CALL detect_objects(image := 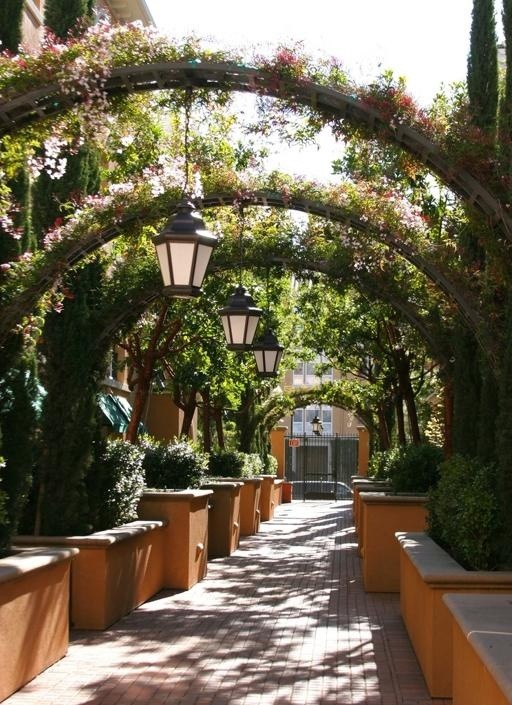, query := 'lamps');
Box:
[250,266,288,379]
[216,198,265,354]
[308,402,325,438]
[149,84,223,300]
